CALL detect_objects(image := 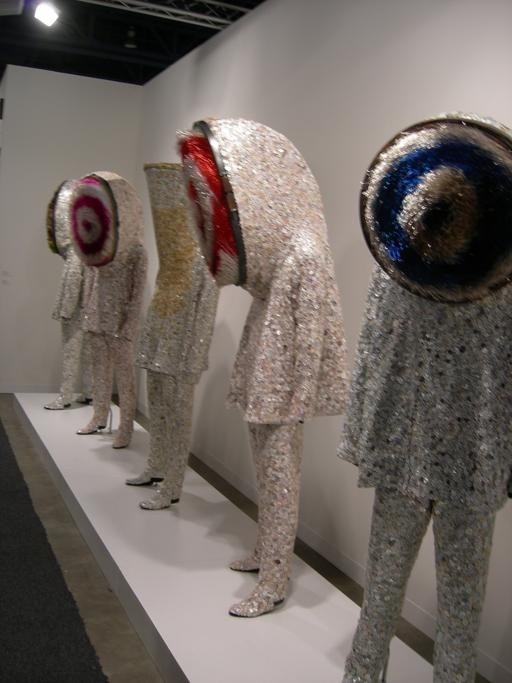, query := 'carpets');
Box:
[0,411,107,683]
